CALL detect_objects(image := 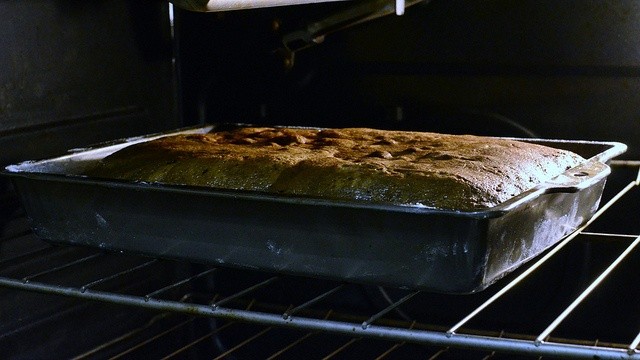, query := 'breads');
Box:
[92,124,587,213]
